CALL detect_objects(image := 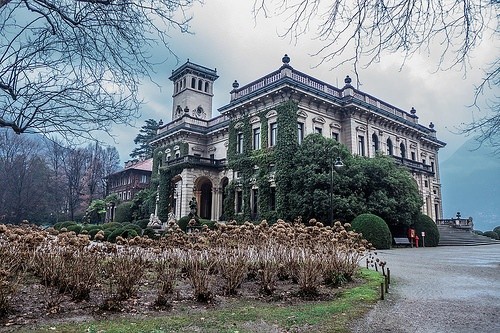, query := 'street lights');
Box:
[328,155,344,227]
